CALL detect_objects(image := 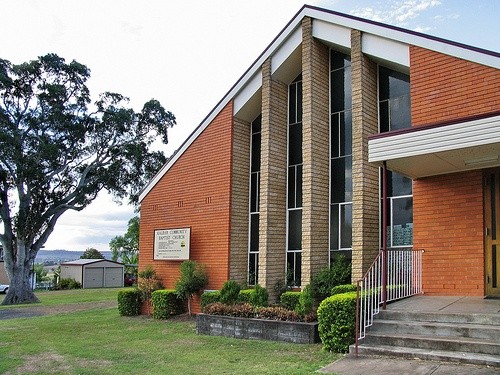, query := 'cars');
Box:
[124,273,135,287]
[0,283,9,295]
